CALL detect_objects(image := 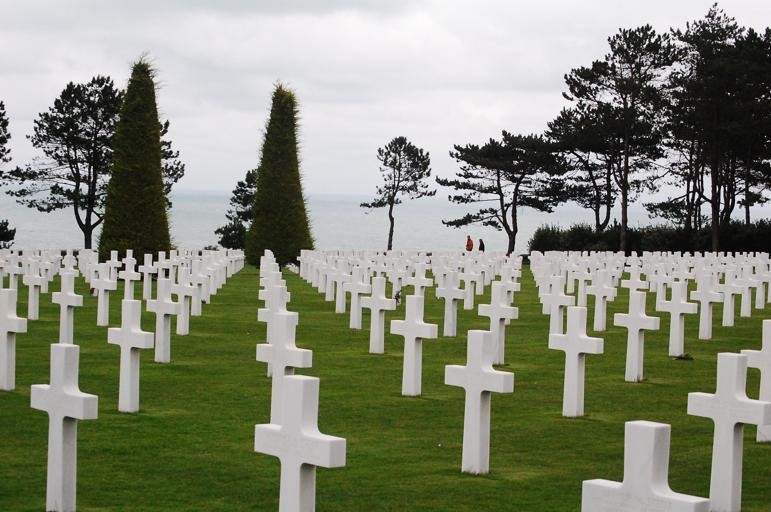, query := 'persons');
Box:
[478,239,484,250]
[465,235,475,251]
